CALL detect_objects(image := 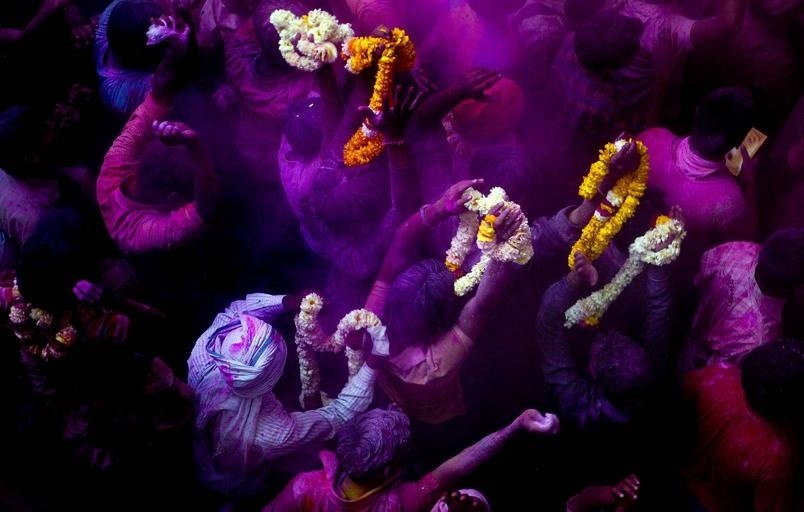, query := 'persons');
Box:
[1,2,802,512]
